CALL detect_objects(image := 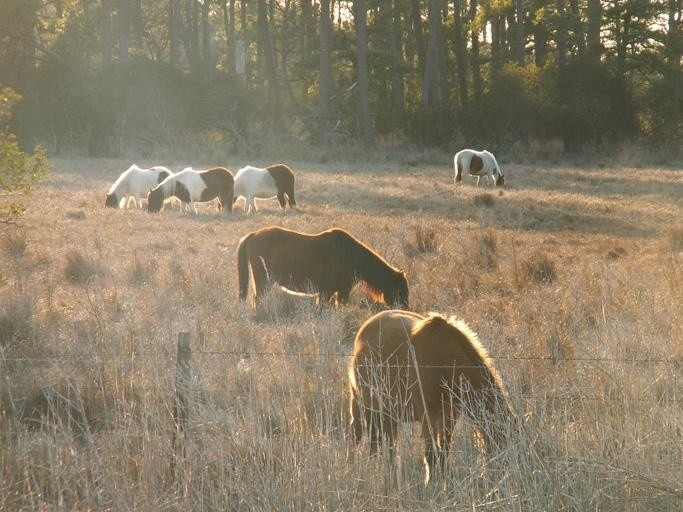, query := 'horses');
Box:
[350,308,533,488]
[104,164,173,209]
[147,167,235,216]
[453,148,505,189]
[218,164,297,215]
[236,225,409,315]
